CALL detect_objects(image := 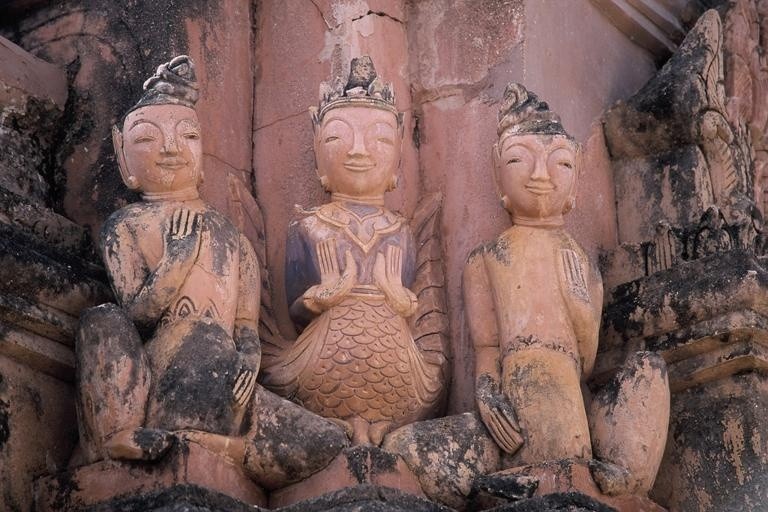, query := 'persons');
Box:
[68,54,265,463]
[455,80,675,499]
[266,50,450,444]
